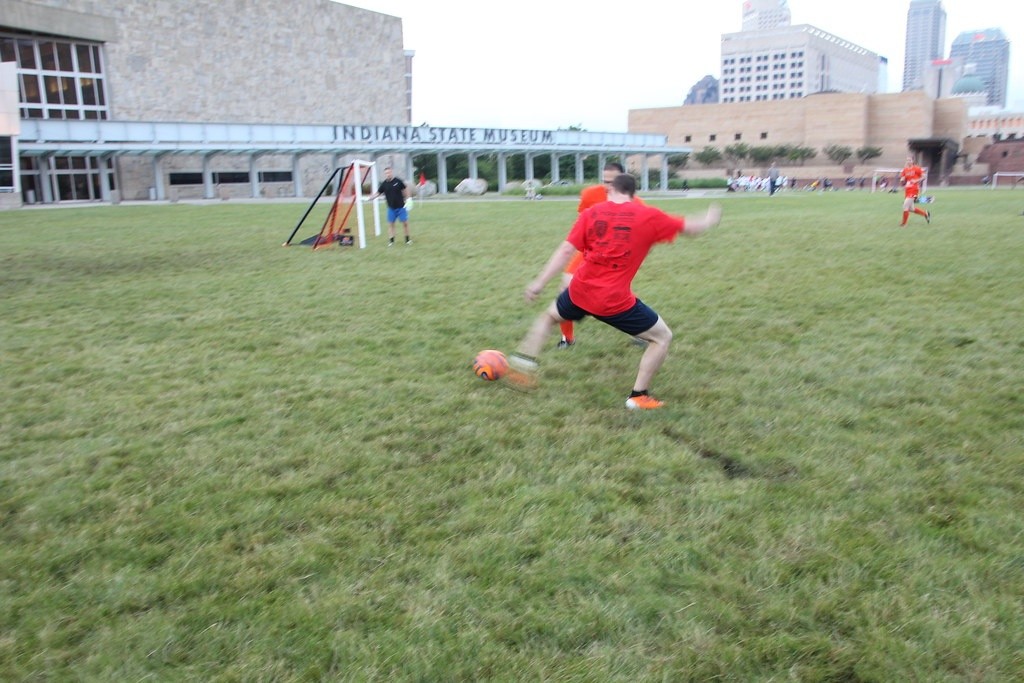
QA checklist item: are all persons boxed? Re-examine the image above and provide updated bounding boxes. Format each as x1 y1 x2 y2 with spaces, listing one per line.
680 161 899 196
365 167 411 245
555 164 645 348
899 156 930 227
508 173 721 410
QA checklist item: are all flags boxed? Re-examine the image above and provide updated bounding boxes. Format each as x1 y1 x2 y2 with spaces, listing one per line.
419 171 426 188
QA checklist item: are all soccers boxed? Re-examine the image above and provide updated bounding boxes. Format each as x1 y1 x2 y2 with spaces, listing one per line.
473 349 509 381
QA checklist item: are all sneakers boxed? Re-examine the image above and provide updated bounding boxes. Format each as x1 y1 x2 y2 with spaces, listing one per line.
552 335 575 352
625 394 665 409
387 242 394 246
405 240 412 245
925 210 930 224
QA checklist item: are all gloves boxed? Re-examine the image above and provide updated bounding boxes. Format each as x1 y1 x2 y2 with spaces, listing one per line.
361 196 370 201
403 197 413 211
